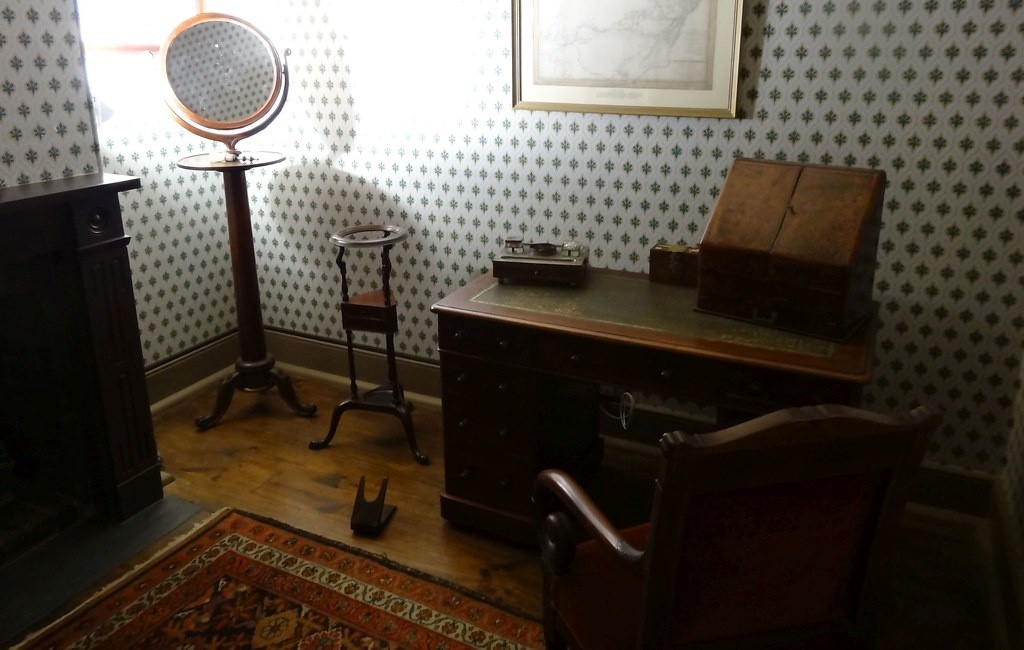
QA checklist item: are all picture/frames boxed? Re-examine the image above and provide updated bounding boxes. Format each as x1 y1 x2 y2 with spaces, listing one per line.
511 0 744 119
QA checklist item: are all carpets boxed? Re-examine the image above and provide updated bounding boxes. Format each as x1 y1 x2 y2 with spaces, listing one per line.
16 508 571 650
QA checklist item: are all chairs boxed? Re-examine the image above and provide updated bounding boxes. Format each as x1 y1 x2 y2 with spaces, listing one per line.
530 403 943 650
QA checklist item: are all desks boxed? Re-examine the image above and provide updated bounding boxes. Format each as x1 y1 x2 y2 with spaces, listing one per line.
177 151 318 430
430 267 873 555
